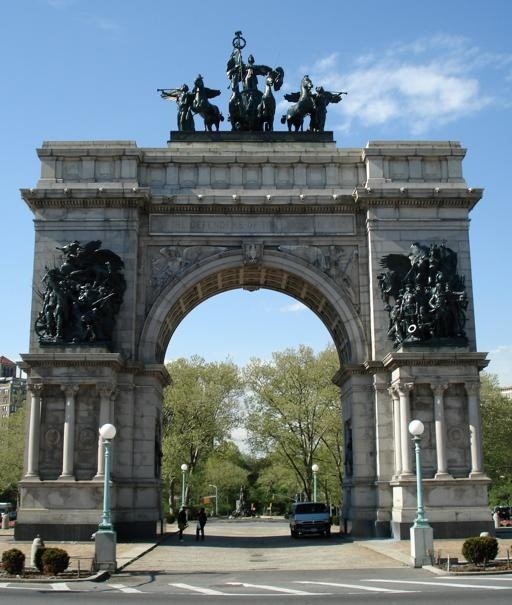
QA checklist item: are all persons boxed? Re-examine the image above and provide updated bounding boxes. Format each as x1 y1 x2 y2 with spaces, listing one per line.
379 238 470 345
163 84 199 129
178 504 189 542
38 239 125 346
245 54 262 90
309 84 339 133
198 507 208 540
493 507 500 527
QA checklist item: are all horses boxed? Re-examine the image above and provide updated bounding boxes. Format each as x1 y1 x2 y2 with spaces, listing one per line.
287 74 317 132
227 74 245 132
191 74 224 131
256 72 276 132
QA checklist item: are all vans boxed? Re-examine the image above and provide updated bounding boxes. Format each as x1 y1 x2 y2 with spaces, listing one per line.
288 502 333 538
0 503 14 521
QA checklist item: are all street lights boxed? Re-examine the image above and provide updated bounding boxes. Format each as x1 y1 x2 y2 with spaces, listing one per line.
311 465 319 503
408 420 431 528
98 423 117 529
209 485 218 516
181 463 188 506
